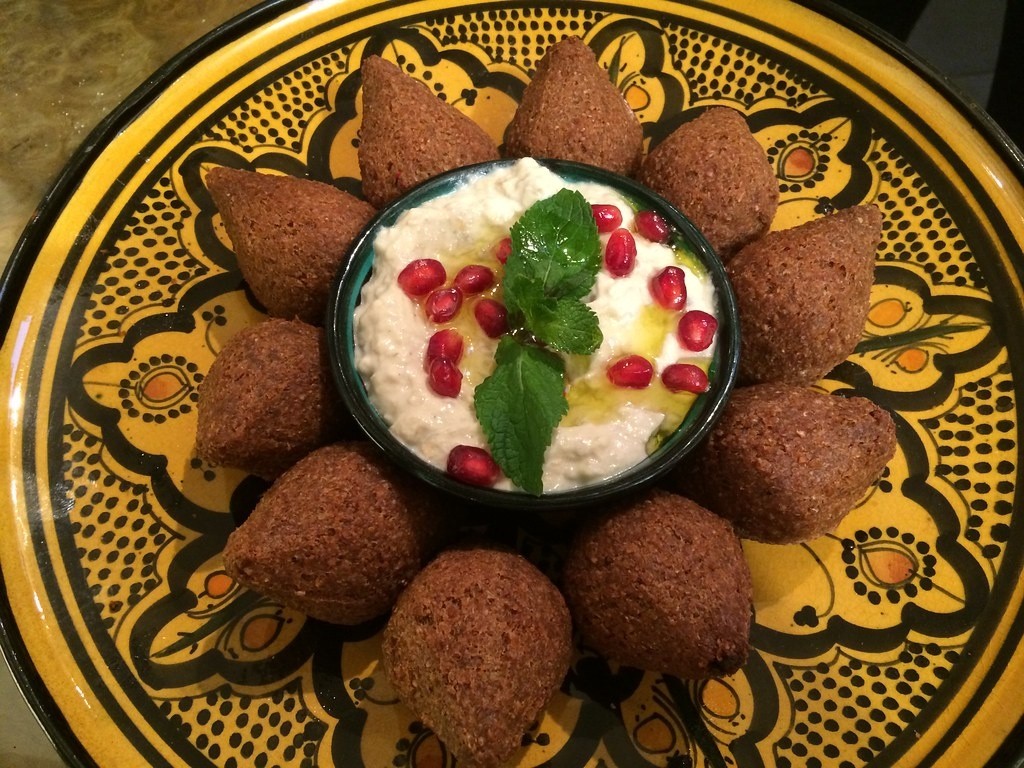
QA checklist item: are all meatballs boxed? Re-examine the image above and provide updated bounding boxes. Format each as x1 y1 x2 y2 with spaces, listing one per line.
638 107 778 256
723 206 882 385
222 437 438 623
196 318 344 474
361 57 499 205
700 382 893 546
204 167 376 318
556 489 747 674
503 38 642 177
382 545 573 767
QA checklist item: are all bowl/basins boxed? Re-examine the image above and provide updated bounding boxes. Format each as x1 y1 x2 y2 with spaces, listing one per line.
322 155 742 541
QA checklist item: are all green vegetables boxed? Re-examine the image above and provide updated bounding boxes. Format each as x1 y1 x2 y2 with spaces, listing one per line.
473 188 604 493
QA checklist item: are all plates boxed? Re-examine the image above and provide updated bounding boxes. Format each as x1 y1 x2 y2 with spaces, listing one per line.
0 1 1024 768
